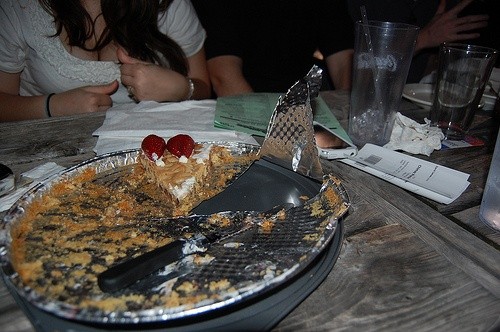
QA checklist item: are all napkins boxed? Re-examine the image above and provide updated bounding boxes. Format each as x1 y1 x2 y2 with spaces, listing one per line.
91 99 262 156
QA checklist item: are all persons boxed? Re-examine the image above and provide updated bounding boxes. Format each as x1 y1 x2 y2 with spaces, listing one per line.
206 0 489 97
0 0 210 123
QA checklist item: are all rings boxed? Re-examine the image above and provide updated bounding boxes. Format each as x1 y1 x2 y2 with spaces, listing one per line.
127 87 133 93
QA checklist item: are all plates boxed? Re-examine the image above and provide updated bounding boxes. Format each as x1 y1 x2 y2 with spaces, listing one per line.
401 83 434 110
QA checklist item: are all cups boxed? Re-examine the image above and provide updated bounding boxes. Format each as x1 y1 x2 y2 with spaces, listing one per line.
430 43 497 140
479 126 500 232
348 21 421 146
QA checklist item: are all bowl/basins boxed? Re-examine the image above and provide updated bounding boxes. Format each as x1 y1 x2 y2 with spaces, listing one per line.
480 93 498 111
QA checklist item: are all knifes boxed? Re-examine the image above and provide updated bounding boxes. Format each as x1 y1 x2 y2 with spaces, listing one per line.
97 203 293 292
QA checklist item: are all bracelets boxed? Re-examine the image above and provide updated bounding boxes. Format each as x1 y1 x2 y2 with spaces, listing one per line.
46 92 56 117
187 79 199 100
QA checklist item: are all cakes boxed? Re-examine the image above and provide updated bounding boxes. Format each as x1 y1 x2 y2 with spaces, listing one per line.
137 134 215 206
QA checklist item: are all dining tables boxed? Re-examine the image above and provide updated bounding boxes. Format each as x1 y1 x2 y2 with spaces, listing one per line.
0 83 500 332
